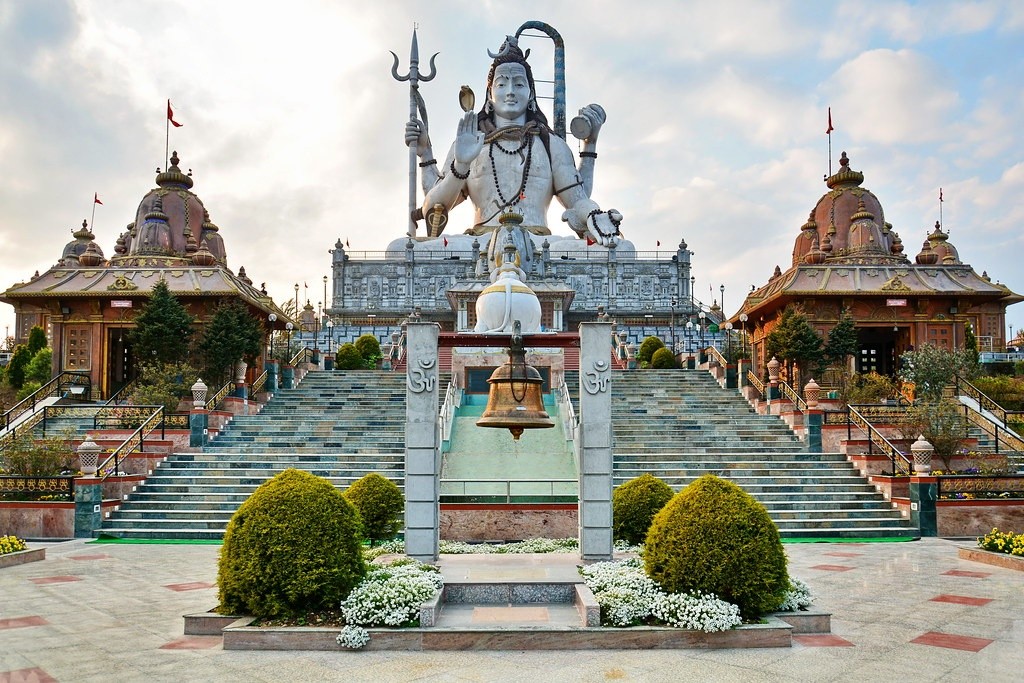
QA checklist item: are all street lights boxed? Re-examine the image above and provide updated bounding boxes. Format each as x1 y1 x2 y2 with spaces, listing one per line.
725 323 732 364
690 276 696 313
739 313 748 360
686 321 693 357
294 283 299 323
323 275 327 316
326 320 332 357
268 313 277 359
286 322 293 365
318 302 322 332
720 285 725 322
699 311 706 348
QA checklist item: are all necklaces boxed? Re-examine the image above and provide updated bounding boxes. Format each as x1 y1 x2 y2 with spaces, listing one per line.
489 134 533 206
493 134 528 155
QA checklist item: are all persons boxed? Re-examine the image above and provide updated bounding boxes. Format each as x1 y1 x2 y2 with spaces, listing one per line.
385 34 635 260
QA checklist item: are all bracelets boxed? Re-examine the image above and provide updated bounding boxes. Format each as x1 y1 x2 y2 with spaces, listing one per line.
587 208 620 237
579 152 597 158
450 159 470 180
419 159 437 167
